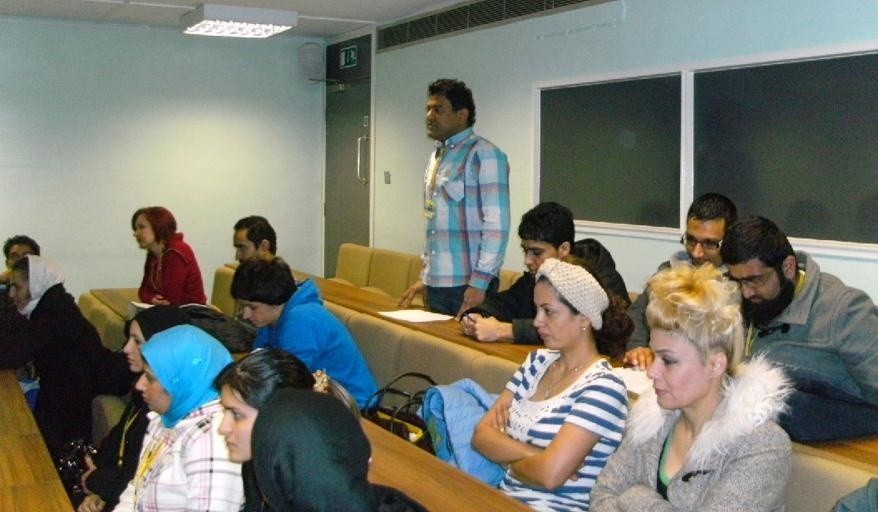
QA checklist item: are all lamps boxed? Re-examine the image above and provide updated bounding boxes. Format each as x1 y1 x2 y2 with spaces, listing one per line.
179 1 298 40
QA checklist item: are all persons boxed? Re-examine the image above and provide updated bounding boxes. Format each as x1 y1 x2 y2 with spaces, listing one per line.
397 79 511 320
458 201 637 354
1 255 111 461
721 217 878 405
590 262 793 512
212 346 360 512
233 215 296 335
472 256 631 512
1 235 40 314
252 387 430 512
131 207 208 306
622 194 738 372
111 325 246 512
75 304 196 512
231 252 380 415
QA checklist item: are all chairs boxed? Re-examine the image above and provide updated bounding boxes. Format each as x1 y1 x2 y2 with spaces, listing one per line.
205 265 238 313
330 241 375 288
354 250 426 297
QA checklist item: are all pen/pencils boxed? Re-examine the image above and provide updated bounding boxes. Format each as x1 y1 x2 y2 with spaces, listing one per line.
464 313 477 324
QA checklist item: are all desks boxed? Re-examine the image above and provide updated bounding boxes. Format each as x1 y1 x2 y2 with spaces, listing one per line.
78 288 538 512
0 357 74 512
225 262 874 494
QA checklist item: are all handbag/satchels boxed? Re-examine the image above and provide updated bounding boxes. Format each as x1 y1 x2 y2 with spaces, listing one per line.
359 371 439 457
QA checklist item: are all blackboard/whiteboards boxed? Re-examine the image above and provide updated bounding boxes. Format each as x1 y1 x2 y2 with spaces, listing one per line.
689 50 878 249
534 68 686 234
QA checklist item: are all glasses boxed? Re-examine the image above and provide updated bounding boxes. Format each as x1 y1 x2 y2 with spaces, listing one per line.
728 268 777 289
679 232 723 250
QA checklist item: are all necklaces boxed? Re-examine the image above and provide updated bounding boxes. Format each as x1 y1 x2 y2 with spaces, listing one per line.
544 355 600 401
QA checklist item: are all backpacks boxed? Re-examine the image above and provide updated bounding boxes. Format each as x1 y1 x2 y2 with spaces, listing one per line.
181 305 254 353
